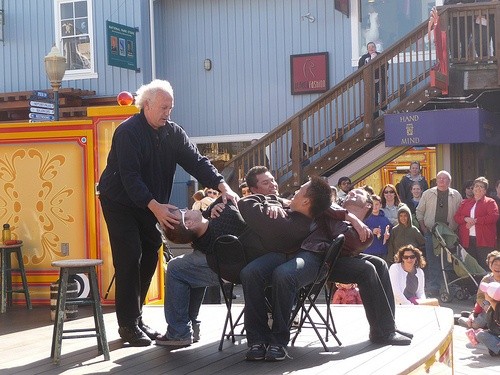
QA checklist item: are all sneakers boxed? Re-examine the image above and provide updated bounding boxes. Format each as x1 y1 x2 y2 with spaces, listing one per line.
246 344 268 361
156 331 194 346
266 345 287 361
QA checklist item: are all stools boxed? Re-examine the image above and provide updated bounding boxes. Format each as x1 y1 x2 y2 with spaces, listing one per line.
0 244 32 313
50 259 110 368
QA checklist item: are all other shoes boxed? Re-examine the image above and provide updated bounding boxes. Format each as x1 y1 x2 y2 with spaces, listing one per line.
118 325 151 345
139 324 159 339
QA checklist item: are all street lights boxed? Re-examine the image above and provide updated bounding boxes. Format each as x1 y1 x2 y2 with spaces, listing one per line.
41 42 68 122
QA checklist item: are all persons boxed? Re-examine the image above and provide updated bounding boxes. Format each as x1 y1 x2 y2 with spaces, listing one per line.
155 166 413 361
127 41 132 55
290 132 317 168
248 139 270 170
98 78 241 343
444 0 496 64
388 161 500 359
358 42 389 119
112 38 117 51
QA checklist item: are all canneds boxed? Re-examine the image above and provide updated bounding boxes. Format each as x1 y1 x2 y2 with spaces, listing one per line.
465 328 479 345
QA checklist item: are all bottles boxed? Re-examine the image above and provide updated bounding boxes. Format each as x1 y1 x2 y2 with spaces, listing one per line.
2 224 11 244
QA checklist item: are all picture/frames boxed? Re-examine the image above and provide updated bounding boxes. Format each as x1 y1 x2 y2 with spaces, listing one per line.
290 52 329 95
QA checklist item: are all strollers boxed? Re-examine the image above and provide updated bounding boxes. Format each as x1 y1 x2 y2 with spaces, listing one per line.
428 220 490 304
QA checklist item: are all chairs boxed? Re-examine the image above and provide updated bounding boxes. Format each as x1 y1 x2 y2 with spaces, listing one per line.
213 234 345 351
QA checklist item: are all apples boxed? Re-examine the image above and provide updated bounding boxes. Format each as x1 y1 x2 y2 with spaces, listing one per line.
117 91 133 105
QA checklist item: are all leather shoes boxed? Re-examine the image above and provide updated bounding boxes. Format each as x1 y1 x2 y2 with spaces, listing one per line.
395 328 414 338
370 331 411 345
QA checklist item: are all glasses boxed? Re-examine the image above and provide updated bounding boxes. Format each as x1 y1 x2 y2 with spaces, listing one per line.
474 184 486 188
242 189 250 194
342 182 350 185
403 254 416 259
384 190 394 194
179 209 189 229
208 191 217 195
